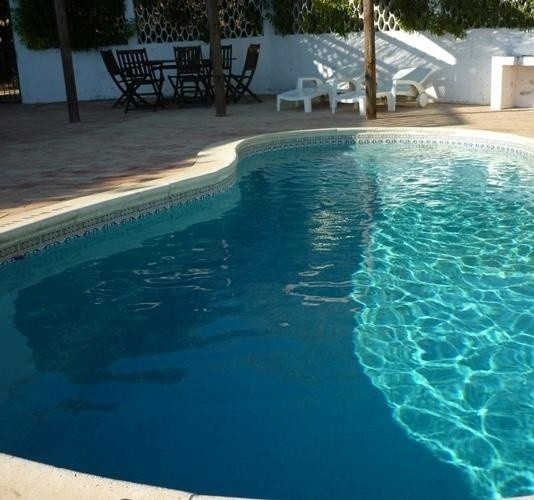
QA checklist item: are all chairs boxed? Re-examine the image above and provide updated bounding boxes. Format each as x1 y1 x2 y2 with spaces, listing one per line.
276 66 436 116
98 43 262 114
